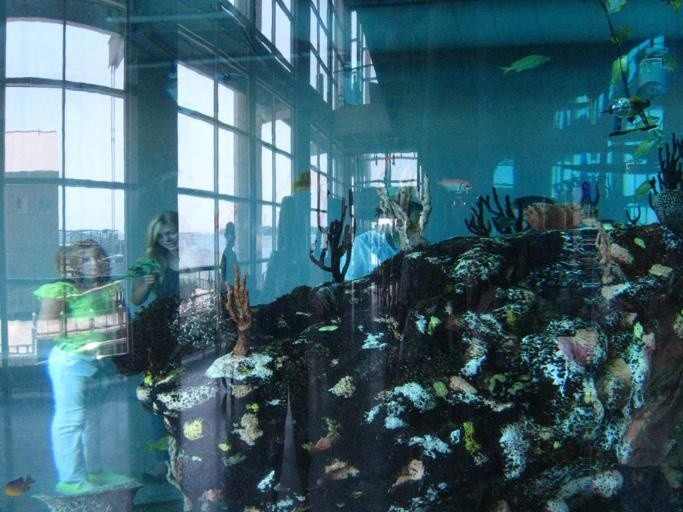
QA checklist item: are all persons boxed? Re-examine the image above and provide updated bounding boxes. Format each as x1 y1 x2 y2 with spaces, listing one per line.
340 195 424 280
221 223 239 281
258 195 310 479
130 212 178 306
33 240 133 495
